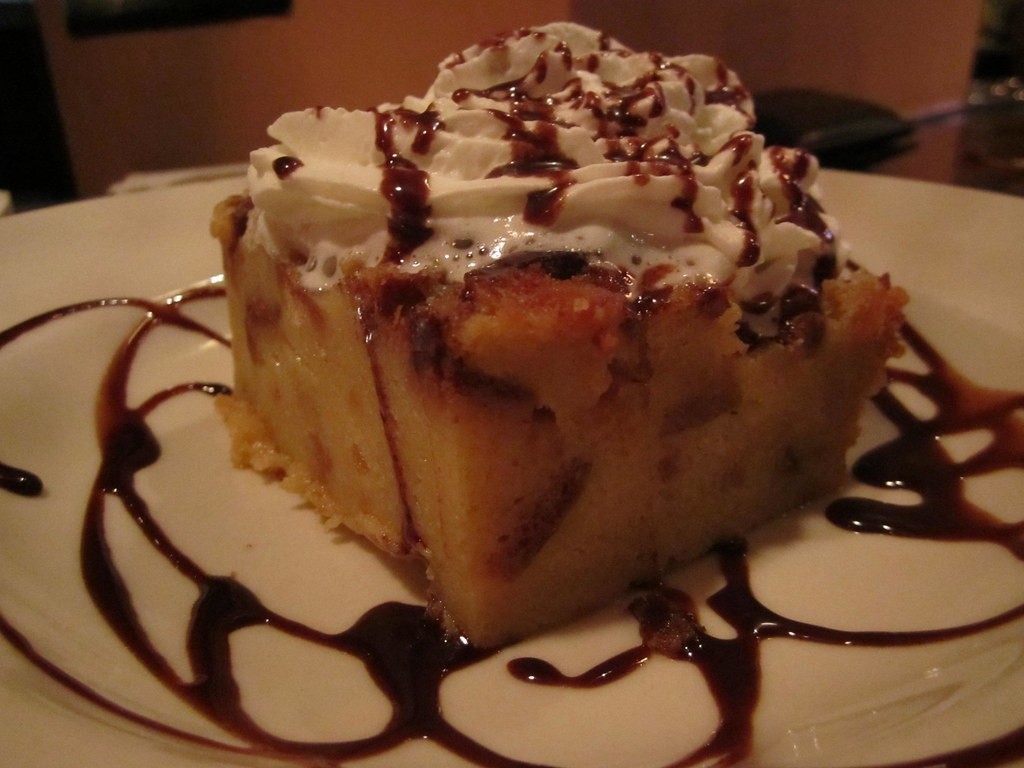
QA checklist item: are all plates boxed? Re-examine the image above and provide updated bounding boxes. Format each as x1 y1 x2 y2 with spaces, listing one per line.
0 170 1024 768
753 86 907 170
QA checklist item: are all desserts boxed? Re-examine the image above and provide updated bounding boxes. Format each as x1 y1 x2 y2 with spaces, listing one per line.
208 24 908 652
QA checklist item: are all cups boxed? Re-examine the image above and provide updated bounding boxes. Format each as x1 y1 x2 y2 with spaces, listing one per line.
0 0 78 215
